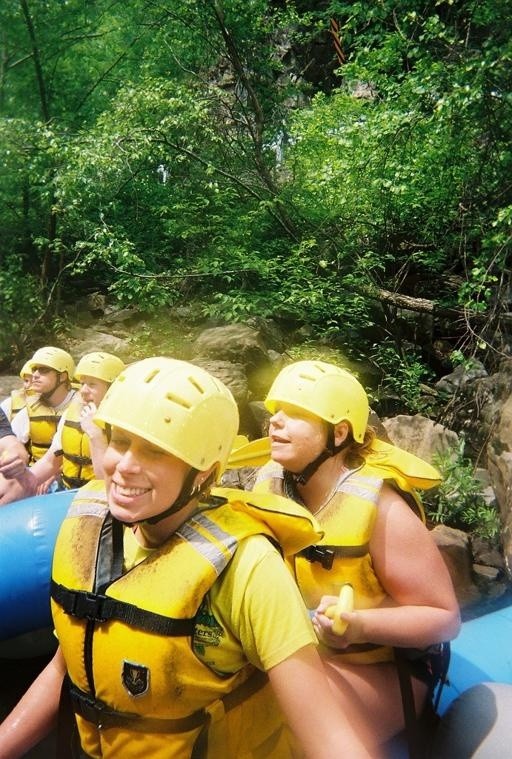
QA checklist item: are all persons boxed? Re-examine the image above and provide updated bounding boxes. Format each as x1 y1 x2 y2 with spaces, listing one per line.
1 405 30 482
244 361 462 758
12 346 80 466
1 358 40 427
0 351 127 507
0 357 373 758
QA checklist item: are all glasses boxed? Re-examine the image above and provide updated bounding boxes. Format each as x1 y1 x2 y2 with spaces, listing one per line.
31 365 52 374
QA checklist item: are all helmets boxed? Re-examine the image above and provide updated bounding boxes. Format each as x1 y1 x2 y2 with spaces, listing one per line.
74 351 124 384
29 346 73 380
263 359 370 448
19 359 31 376
95 355 241 484
